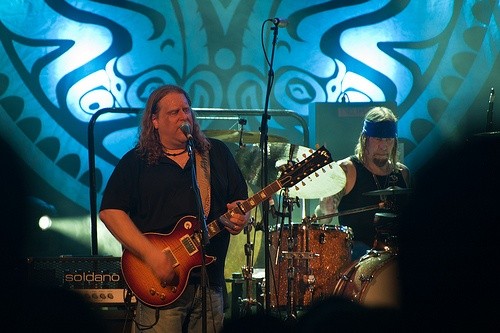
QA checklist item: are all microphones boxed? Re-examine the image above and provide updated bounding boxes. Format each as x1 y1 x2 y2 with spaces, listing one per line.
180 122 193 140
270 17 288 27
487 87 495 132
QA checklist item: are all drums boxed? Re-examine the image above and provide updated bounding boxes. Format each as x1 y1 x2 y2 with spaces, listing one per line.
334 250 397 307
269 223 354 312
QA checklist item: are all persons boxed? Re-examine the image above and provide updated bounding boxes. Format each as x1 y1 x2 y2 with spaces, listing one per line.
99 84 251 333
314 106 409 263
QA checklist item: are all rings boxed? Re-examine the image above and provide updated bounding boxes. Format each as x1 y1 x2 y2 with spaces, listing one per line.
234 226 236 229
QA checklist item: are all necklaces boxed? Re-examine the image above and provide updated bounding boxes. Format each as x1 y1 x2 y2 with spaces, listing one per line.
164 149 186 156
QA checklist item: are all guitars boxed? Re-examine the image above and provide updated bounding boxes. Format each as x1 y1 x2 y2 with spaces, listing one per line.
120 144 334 308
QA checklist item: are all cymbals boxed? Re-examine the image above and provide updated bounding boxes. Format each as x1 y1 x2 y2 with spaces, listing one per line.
362 186 411 196
201 130 289 143
234 144 347 199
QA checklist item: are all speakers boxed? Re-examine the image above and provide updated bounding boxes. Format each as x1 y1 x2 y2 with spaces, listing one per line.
308 101 397 162
